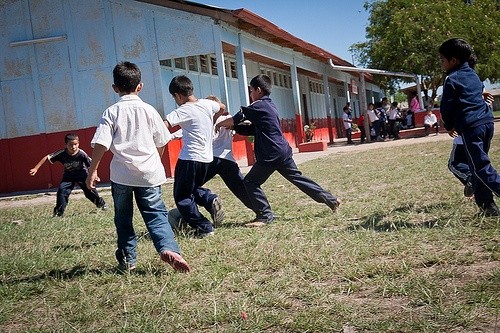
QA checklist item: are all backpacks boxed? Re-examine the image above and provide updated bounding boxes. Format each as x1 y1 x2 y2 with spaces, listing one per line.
375 108 387 124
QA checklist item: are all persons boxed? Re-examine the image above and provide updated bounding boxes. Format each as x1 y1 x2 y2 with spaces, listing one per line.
202 96 266 222
343 102 366 144
163 75 225 237
411 89 441 111
438 38 500 217
30 134 109 217
424 109 439 136
367 97 413 141
86 61 192 272
215 74 340 227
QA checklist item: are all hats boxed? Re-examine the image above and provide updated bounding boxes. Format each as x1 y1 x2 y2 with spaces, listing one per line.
381 97 389 102
392 102 398 107
425 108 433 112
368 103 375 107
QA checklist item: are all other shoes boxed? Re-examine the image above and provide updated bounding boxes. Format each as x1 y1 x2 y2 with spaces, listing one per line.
464 181 474 196
245 221 268 226
371 135 398 141
102 203 109 211
210 197 225 228
348 141 354 144
192 230 217 239
117 262 136 272
332 198 343 214
160 250 191 274
473 202 500 222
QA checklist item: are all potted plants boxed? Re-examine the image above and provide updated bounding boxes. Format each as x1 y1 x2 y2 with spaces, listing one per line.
299 120 327 152
351 123 362 139
